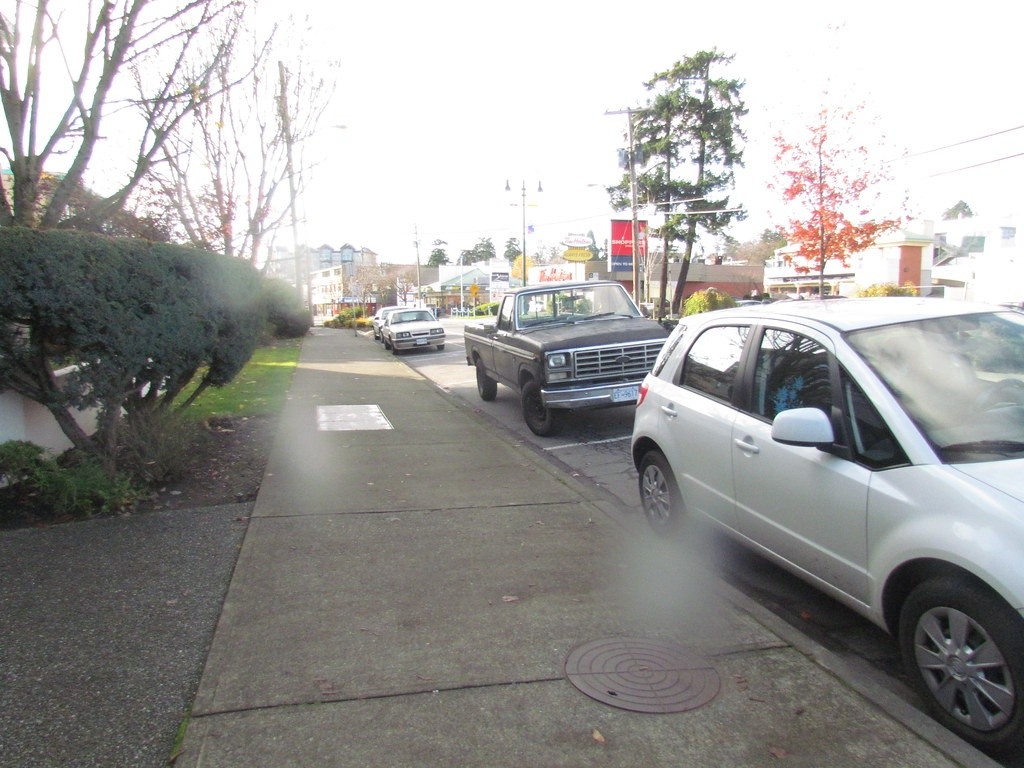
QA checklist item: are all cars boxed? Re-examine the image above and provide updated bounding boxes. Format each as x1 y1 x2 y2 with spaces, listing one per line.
372 306 409 340
381 308 447 355
639 297 670 316
735 300 762 307
631 296 1024 750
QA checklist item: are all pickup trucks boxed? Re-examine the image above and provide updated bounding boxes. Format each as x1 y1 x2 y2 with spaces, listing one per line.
464 280 670 436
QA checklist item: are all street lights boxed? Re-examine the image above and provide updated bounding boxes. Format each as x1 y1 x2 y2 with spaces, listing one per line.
505 179 543 315
